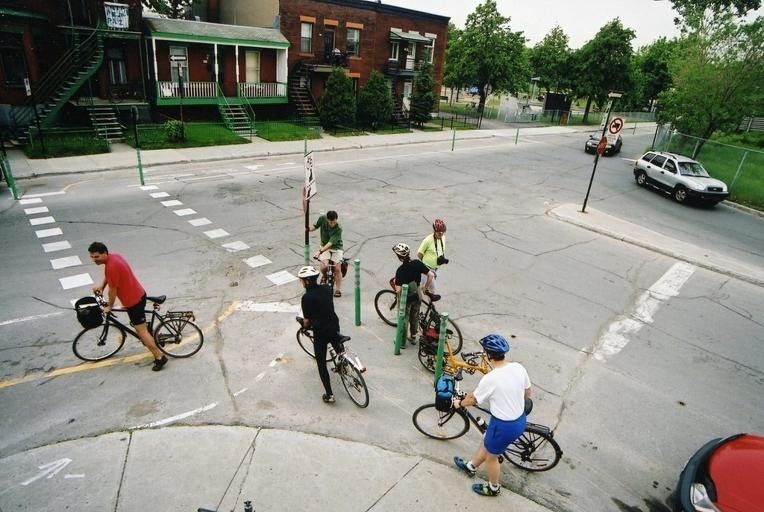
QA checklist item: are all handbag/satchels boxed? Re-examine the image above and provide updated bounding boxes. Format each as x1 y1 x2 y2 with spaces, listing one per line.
437 375 453 398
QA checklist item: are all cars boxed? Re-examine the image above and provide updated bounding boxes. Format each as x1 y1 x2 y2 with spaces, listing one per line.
676 432 764 512
585 129 623 156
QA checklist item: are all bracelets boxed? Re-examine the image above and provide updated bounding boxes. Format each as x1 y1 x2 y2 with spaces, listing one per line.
458 399 463 407
318 249 323 255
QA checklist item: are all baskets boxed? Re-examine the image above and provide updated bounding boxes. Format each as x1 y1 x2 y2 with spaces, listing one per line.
435 374 453 411
75 296 103 329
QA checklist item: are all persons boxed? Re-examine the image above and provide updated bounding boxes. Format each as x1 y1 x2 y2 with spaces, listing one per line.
454 334 533 497
88 242 168 371
416 219 447 295
296 265 347 403
308 211 345 297
392 243 434 349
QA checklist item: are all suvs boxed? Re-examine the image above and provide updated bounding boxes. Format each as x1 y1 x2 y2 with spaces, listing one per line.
633 150 729 206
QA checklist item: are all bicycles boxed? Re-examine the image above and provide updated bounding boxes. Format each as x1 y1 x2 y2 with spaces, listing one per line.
416 325 493 380
374 275 463 360
412 372 562 473
294 313 370 409
71 287 205 363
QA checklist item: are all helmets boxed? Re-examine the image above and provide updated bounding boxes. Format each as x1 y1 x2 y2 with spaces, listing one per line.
479 334 509 353
392 243 410 258
297 266 320 279
433 218 446 233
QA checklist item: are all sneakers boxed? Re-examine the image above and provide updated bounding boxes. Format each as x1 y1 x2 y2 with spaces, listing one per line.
408 336 416 345
333 291 341 297
323 393 336 402
472 482 501 496
152 355 168 371
394 338 406 349
454 456 476 477
